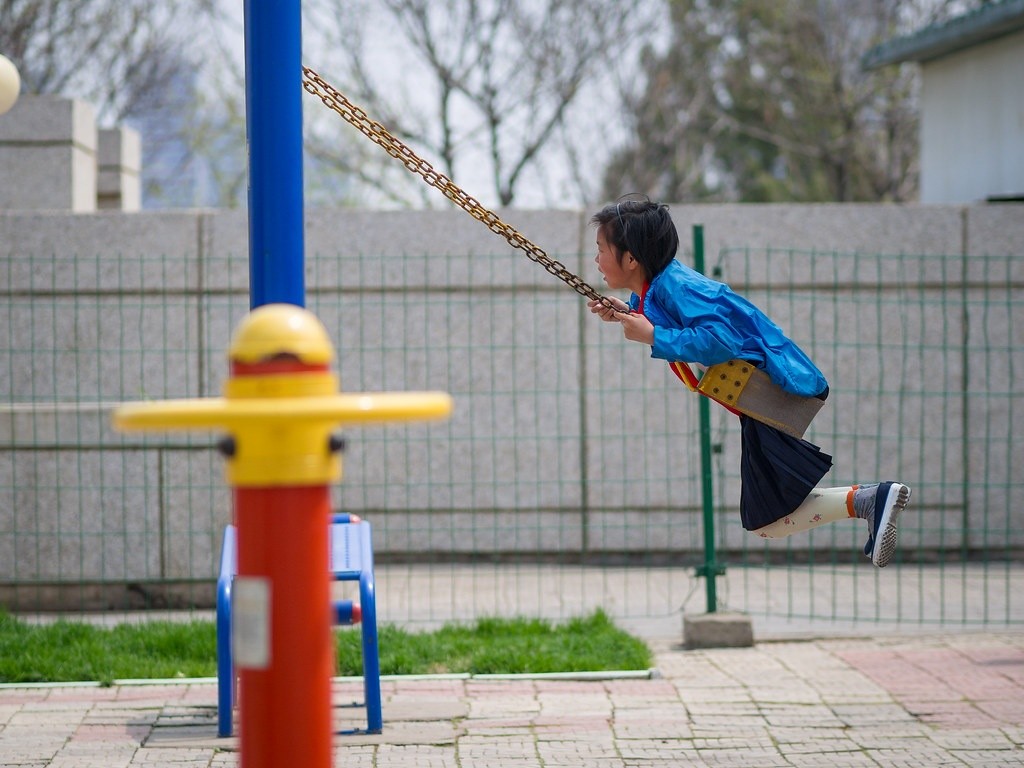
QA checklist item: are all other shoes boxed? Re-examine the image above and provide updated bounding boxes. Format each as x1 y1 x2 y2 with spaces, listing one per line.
864 481 912 558
870 483 908 568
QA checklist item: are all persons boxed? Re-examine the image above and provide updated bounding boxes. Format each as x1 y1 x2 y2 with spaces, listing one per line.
586 192 912 568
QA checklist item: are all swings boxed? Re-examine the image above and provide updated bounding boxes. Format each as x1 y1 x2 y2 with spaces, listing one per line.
303 65 824 441
